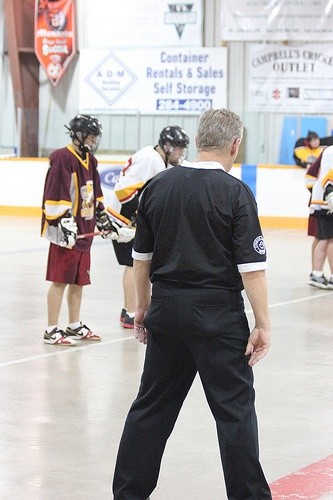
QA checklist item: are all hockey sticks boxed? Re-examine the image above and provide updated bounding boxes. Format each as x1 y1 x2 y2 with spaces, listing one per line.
114 220 136 244
43 224 109 247
325 191 333 214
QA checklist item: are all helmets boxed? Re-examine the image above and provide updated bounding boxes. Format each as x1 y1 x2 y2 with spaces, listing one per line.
64 114 103 142
159 124 190 147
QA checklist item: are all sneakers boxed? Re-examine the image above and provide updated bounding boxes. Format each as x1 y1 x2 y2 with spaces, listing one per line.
66 324 102 341
308 272 333 290
43 327 77 346
120 308 135 329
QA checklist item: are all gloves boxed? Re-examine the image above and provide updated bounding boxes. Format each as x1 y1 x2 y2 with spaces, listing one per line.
59 217 78 246
96 210 121 240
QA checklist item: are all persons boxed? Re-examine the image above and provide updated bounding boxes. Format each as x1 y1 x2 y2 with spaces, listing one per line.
303 144 333 289
112 108 272 500
40 115 100 346
293 130 333 169
105 125 190 328
